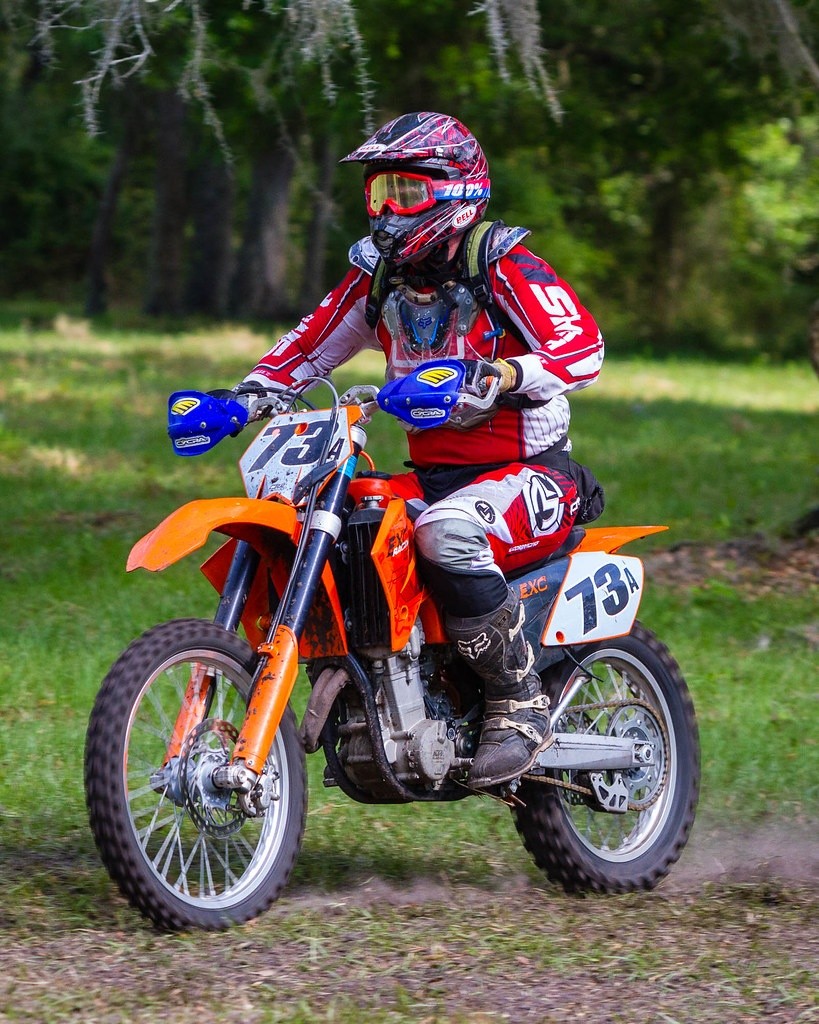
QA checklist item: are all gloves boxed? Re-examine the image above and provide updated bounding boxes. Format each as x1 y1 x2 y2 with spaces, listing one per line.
456 357 513 397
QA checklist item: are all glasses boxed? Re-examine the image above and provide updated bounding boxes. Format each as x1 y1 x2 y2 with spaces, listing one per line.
366 173 437 215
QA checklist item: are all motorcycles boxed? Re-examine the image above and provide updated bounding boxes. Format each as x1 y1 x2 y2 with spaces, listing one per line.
83 360 701 935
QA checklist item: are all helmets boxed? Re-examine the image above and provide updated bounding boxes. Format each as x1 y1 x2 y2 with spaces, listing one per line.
339 112 489 267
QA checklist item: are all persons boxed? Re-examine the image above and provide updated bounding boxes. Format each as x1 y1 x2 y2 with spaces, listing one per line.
163 108 609 791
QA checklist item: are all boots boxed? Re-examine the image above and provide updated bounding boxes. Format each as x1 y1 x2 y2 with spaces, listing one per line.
322 733 342 786
440 584 558 790
